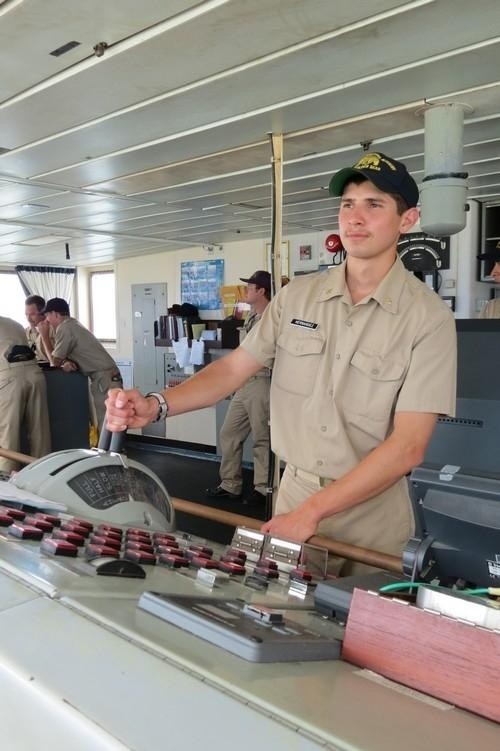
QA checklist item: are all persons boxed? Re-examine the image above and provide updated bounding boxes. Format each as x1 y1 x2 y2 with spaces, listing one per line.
104 151 460 576
0 316 52 477
205 271 275 505
35 298 126 455
475 239 500 319
23 294 58 361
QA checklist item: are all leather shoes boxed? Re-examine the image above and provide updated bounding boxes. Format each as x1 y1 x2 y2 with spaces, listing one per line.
201 485 234 497
245 490 265 501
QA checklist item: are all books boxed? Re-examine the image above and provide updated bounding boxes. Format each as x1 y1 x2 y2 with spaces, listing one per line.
159 312 206 342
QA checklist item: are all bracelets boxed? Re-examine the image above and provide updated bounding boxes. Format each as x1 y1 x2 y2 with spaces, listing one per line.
145 392 168 423
61 359 68 364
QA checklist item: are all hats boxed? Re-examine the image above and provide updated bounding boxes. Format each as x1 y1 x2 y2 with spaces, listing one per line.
40 298 69 314
329 151 420 212
240 270 271 289
